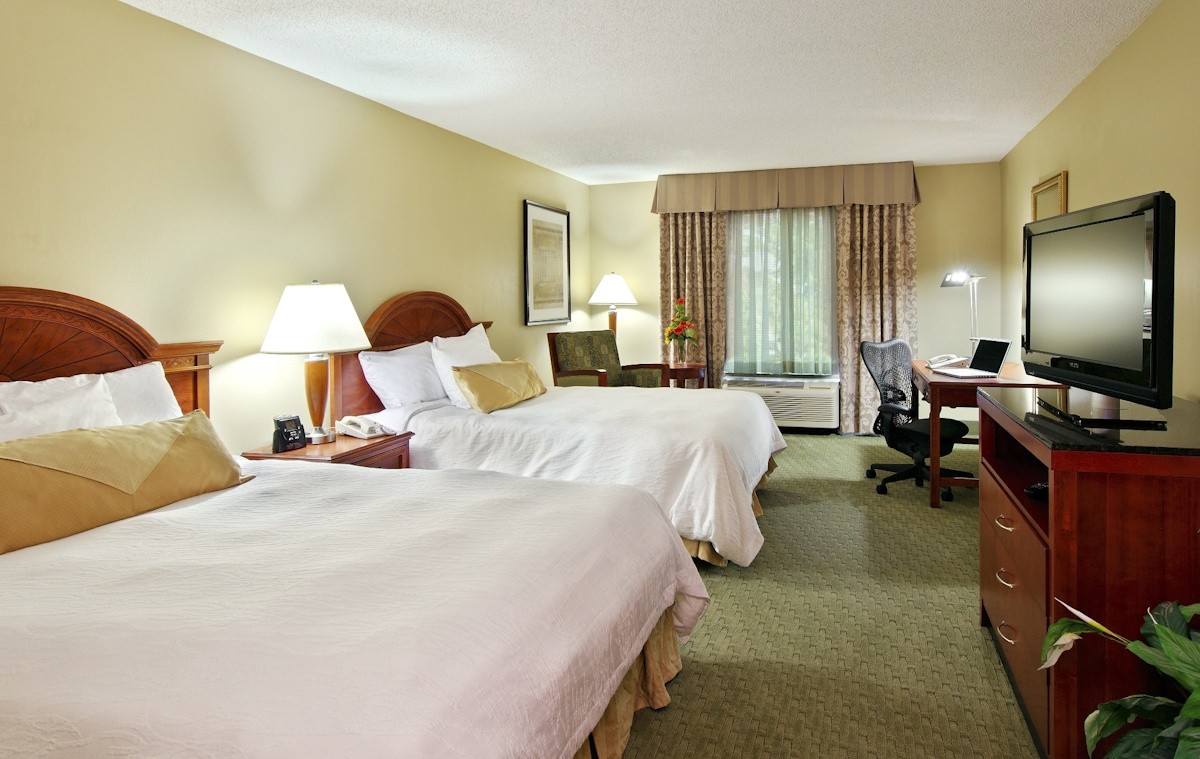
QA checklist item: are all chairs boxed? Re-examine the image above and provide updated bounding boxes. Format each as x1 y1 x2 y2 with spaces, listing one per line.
861 337 977 502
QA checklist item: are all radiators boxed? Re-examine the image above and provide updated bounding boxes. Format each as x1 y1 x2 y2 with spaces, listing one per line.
721 372 840 436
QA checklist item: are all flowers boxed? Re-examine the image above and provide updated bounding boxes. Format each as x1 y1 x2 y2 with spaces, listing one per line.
664 297 701 363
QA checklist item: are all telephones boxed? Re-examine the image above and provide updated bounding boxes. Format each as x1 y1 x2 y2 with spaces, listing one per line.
923 354 969 371
334 415 397 441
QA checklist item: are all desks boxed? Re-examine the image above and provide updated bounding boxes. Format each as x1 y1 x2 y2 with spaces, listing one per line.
668 362 707 389
910 358 1071 509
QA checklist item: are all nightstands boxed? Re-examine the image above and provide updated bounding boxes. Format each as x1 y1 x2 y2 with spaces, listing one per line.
240 430 415 469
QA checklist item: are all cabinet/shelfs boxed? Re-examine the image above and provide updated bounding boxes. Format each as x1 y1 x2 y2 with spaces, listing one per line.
974 390 1200 759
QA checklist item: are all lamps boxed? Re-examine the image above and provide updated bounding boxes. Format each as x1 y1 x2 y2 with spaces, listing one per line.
941 270 986 356
258 280 372 445
587 272 639 338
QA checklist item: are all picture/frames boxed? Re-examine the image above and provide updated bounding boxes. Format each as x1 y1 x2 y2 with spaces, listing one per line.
1031 170 1068 221
523 199 572 326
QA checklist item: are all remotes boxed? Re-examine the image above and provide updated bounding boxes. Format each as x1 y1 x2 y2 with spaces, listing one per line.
1024 479 1049 498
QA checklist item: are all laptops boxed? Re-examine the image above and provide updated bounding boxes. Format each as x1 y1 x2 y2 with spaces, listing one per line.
933 336 1011 378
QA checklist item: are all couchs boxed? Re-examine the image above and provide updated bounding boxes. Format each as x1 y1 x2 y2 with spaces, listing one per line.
547 329 670 388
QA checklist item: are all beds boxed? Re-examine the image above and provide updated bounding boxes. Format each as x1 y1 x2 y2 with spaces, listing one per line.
1 453 712 759
356 384 789 570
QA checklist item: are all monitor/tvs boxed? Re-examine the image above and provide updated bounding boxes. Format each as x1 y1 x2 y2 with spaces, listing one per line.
1022 190 1176 409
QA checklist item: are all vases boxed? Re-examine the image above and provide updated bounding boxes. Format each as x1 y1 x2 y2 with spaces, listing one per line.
676 338 687 368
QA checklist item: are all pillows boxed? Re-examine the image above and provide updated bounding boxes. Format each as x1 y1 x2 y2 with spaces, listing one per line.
0 408 256 555
451 357 547 414
103 361 184 424
431 322 503 410
358 341 448 409
0 373 122 440
